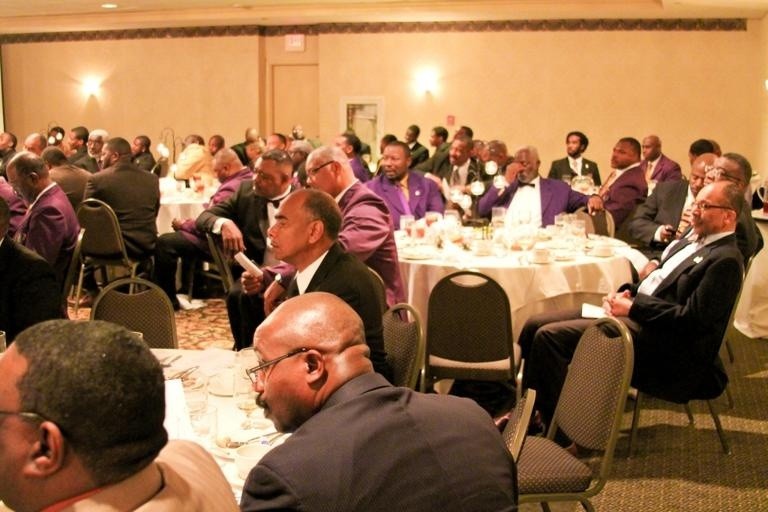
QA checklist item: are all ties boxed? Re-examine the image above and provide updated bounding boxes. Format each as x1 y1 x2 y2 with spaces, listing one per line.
286 279 298 297
393 180 411 215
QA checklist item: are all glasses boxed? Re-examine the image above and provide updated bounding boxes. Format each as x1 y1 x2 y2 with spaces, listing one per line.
704 166 743 182
692 201 731 211
309 159 333 174
246 347 308 384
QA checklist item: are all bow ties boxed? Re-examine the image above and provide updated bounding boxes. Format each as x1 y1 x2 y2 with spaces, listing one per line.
266 198 279 208
518 183 535 187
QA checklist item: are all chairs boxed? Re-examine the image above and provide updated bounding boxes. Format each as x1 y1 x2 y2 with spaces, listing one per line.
573 206 615 239
382 303 424 389
369 266 387 314
205 232 235 295
89 278 178 351
719 252 755 362
629 387 734 455
502 389 537 464
512 316 634 512
74 198 154 319
425 271 525 419
63 228 86 298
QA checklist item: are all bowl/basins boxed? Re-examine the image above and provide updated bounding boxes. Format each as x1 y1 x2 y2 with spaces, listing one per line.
187 347 275 477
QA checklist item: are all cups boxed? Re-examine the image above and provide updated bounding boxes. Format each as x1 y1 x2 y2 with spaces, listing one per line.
561 175 599 195
176 174 205 200
401 206 614 265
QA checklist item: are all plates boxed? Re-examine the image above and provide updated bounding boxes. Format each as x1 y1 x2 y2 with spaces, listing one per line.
165 371 203 390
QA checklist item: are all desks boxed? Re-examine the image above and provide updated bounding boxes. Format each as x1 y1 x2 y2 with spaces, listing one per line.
155 175 222 237
749 207 768 229
151 347 293 504
392 228 649 342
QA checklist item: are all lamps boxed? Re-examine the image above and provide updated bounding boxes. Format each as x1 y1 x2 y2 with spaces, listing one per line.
39 119 63 149
154 126 187 166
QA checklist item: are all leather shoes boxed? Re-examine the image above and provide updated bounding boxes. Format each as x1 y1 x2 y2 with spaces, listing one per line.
494 408 543 432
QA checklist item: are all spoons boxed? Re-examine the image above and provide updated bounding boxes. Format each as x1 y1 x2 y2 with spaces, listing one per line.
160 354 183 367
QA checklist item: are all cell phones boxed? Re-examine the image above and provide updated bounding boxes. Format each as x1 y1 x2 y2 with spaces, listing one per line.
666 227 676 237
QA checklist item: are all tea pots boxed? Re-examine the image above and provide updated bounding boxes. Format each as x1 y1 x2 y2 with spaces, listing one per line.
757 179 768 217
